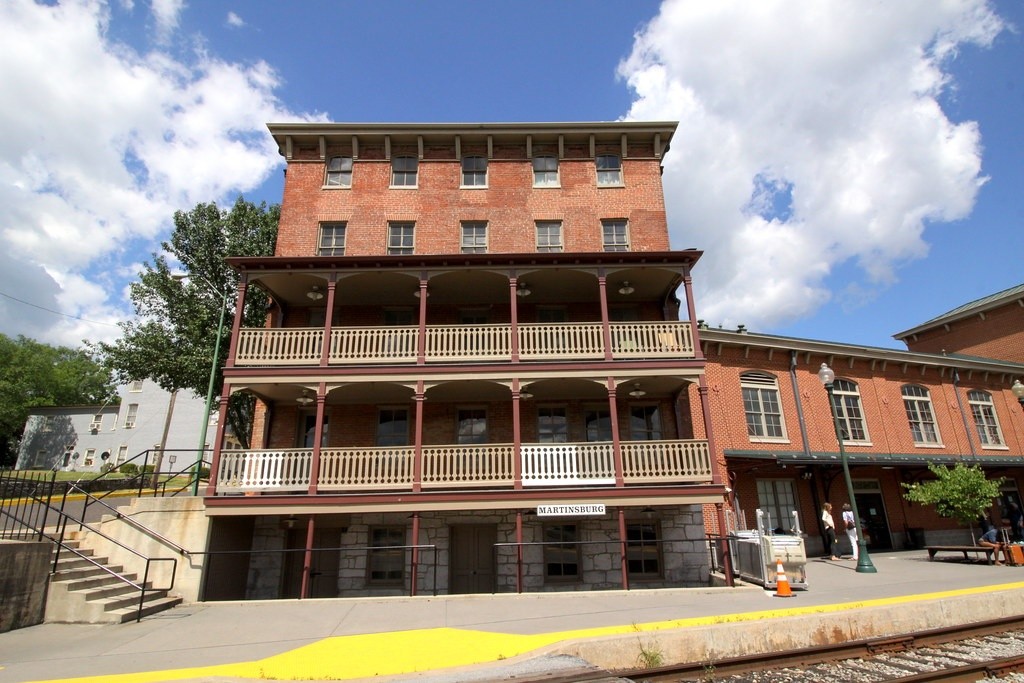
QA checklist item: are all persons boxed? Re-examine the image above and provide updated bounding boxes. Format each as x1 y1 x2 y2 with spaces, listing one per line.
821 501 859 561
977 522 1007 567
999 501 1024 541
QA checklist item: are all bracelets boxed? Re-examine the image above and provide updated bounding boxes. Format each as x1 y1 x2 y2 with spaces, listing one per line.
999 541 1001 545
1003 542 1004 544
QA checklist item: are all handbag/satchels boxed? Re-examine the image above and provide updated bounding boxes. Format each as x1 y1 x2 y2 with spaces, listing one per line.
848 521 854 530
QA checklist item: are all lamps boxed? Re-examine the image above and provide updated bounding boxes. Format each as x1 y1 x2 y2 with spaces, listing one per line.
410 396 428 400
641 507 655 516
296 390 313 405
306 286 324 300
524 510 537 516
618 281 635 295
516 283 531 296
285 515 298 527
414 286 430 297
407 514 422 519
629 383 646 399
520 385 533 401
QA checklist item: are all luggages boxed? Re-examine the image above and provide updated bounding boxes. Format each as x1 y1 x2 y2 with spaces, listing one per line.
1001 528 1024 567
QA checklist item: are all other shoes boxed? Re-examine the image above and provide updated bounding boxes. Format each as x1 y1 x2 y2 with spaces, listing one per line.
848 557 857 561
829 555 841 561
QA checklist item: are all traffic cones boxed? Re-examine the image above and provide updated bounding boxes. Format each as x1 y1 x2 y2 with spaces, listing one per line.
770 559 799 597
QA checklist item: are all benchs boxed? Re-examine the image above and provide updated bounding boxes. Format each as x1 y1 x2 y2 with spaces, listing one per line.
922 545 996 564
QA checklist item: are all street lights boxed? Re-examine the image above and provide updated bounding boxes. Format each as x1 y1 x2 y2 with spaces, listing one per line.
816 362 877 574
